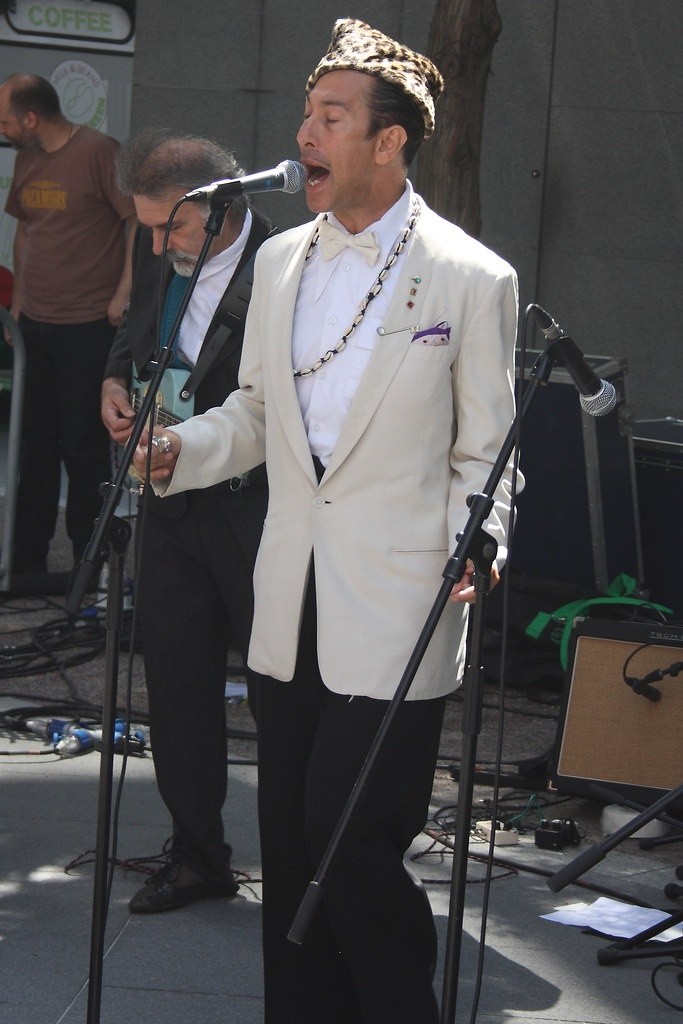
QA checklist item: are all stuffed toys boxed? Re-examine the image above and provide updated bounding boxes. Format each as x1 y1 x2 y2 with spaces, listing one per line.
412 321 451 345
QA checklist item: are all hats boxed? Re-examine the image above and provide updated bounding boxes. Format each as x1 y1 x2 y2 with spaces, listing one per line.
306 17 446 141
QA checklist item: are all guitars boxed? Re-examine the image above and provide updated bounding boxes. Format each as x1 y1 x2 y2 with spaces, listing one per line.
111 359 249 517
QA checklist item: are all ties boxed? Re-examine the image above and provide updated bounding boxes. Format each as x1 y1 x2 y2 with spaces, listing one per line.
160 273 195 371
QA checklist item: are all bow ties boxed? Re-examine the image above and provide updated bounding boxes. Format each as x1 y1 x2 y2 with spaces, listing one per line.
319 221 379 267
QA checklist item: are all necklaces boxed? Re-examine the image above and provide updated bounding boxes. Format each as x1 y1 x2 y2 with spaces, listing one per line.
292 193 421 378
68 123 72 140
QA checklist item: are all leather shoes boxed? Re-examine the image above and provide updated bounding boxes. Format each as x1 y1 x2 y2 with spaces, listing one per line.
128 847 236 914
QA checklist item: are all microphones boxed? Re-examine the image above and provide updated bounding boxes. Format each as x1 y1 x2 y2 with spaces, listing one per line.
184 160 307 202
625 676 662 702
532 304 617 417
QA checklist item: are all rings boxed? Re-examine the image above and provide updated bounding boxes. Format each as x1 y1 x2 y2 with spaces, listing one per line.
153 436 172 453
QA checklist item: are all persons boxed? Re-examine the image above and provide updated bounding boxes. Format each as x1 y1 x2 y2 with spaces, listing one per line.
0 263 15 369
0 75 138 574
101 128 279 914
131 18 525 1024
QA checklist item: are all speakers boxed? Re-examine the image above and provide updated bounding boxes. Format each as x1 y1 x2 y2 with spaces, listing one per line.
547 417 683 814
